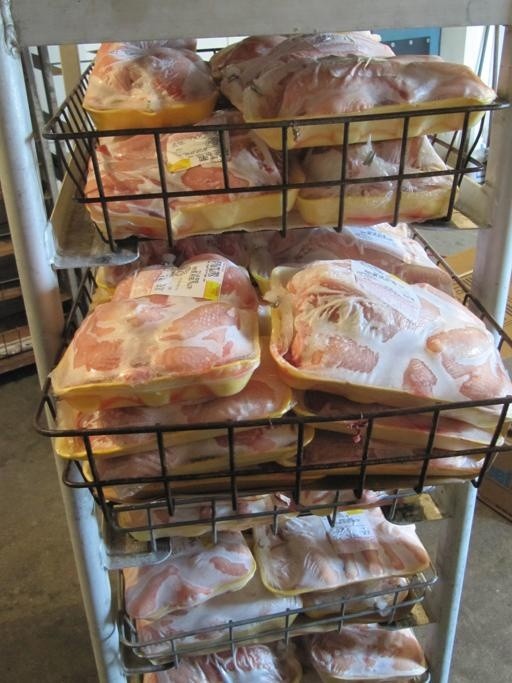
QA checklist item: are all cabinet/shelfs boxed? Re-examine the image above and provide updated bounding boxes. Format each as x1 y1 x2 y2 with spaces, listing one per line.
0 0 511 681
